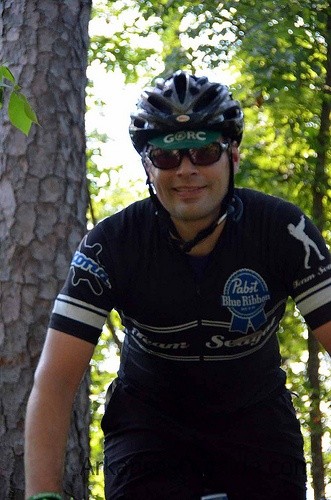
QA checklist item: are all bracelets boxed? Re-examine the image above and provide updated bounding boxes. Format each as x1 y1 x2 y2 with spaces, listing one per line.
23 490 64 499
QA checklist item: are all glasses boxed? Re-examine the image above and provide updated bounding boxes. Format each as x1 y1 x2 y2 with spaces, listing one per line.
140 142 229 170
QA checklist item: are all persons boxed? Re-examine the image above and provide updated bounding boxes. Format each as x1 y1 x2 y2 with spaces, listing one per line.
20 74 331 499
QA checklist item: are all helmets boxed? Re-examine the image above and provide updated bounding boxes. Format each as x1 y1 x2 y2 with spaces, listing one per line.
128 71 244 156
